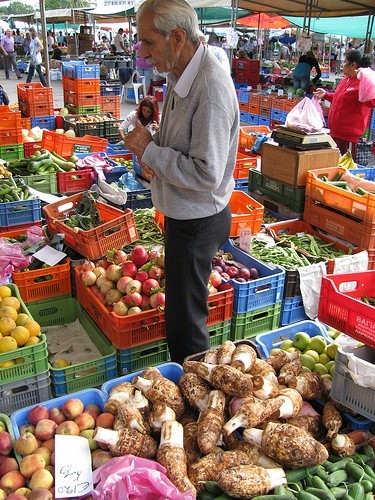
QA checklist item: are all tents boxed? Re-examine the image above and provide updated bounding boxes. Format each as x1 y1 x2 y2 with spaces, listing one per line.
0 0 375 60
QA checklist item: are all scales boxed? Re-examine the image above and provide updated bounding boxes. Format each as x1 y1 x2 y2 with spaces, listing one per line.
272 125 338 151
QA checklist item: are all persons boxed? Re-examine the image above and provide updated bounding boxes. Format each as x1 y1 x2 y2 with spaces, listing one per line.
314 50 375 163
25 28 47 88
0 85 9 106
124 0 239 365
95 28 153 98
207 33 375 74
118 96 162 141
0 28 70 80
292 51 320 93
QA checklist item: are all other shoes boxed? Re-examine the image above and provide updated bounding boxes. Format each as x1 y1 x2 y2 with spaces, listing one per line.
18 76 23 79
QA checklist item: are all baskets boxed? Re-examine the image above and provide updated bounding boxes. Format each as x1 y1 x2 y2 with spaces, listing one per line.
0 53 374 473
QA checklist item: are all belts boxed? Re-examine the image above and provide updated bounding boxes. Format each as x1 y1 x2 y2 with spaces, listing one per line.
6 51 14 53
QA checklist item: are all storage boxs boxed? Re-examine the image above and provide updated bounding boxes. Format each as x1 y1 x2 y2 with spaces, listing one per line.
1 57 375 488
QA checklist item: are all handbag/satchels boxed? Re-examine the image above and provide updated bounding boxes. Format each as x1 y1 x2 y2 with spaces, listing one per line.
30 53 37 67
309 94 327 127
284 96 323 134
355 67 375 102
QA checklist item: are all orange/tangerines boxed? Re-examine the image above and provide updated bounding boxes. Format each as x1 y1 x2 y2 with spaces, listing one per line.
55 359 69 368
0 285 41 369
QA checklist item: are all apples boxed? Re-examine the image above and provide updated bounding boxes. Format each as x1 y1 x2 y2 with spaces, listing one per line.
269 331 338 379
208 257 259 295
0 397 116 500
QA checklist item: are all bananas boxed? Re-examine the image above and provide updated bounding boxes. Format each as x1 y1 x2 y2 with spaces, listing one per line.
338 150 354 170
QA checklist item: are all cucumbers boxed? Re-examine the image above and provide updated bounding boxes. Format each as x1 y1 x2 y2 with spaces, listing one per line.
320 171 369 196
0 174 36 212
194 444 375 500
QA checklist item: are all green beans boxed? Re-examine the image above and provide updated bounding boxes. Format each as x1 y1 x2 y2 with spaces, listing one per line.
132 207 165 246
248 233 352 271
